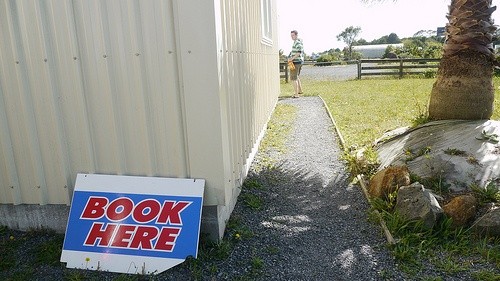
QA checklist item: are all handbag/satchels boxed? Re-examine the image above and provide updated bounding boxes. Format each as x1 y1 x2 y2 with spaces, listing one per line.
287 59 295 72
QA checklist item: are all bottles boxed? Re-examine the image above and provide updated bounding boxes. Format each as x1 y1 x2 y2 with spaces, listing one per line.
288 61 295 70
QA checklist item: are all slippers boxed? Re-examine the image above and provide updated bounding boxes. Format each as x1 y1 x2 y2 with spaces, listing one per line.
291 91 303 98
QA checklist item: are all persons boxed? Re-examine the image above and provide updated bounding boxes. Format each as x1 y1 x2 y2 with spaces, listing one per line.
287 31 305 98
